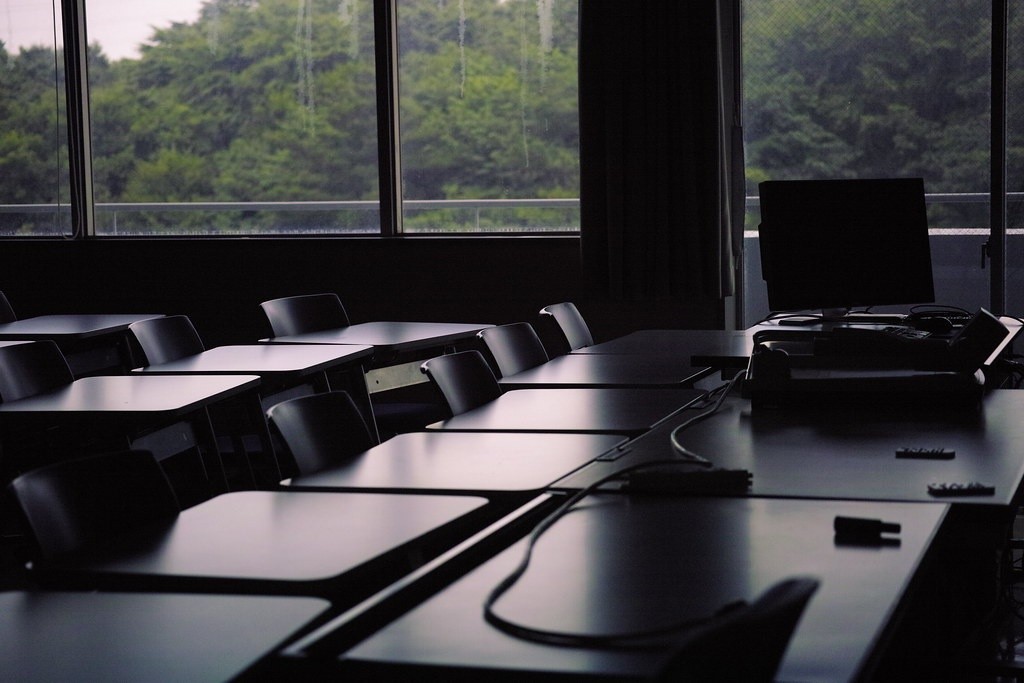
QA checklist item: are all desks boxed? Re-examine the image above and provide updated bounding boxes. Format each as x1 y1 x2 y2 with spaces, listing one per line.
748 310 1024 387
425 387 708 432
260 321 497 443
552 387 1024 683
0 590 331 683
0 375 261 493
568 329 757 358
0 314 166 376
132 344 380 488
341 494 952 683
279 431 630 494
497 355 712 384
56 491 489 584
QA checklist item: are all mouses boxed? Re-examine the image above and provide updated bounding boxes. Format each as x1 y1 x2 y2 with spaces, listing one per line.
925 317 954 334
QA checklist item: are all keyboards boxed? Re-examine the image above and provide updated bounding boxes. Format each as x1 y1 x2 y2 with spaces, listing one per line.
880 327 932 342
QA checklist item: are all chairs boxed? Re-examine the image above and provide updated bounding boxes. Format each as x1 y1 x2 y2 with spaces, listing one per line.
259 294 434 404
3 451 184 561
420 351 503 418
0 340 74 403
475 322 549 377
263 390 375 480
127 315 276 488
539 301 594 352
0 290 16 326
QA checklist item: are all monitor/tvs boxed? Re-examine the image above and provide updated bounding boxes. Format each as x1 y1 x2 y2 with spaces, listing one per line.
757 177 935 326
